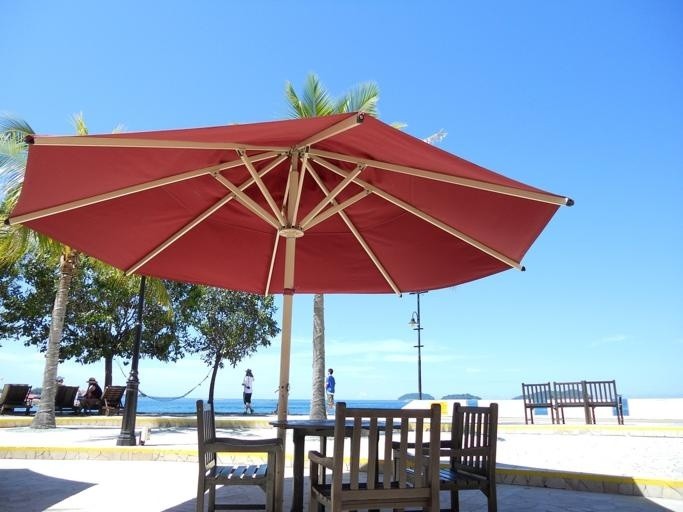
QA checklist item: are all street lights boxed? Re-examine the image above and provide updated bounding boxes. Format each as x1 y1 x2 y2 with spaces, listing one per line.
409 292 424 399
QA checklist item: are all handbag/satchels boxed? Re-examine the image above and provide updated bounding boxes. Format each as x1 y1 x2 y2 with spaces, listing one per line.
73 391 81 408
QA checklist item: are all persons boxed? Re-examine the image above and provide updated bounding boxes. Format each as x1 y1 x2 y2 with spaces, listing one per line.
57 375 65 385
71 376 102 416
242 368 254 414
324 368 336 409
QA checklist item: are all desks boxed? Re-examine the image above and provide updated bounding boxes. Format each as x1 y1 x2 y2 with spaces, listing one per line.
269 419 409 510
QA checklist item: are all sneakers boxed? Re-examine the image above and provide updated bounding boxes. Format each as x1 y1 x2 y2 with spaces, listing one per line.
243 410 247 414
250 409 254 414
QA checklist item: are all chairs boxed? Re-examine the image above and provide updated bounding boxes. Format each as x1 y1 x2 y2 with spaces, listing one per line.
391 402 500 512
195 397 286 512
308 400 441 511
0 384 33 416
582 379 625 425
53 384 79 414
552 381 590 424
78 385 127 415
521 382 557 425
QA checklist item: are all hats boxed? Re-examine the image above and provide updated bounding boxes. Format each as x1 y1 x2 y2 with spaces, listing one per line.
57 376 64 381
245 369 252 374
85 377 98 384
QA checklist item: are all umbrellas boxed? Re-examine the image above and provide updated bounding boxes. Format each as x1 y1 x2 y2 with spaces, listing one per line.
3 110 575 511
